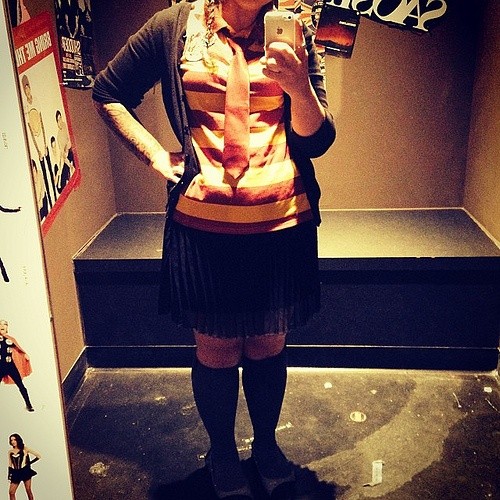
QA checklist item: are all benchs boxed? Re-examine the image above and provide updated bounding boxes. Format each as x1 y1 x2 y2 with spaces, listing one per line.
70 206 500 372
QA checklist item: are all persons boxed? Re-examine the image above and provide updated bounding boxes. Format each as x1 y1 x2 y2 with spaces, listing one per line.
8 434 41 500
21 75 76 220
1 319 35 412
90 0 338 500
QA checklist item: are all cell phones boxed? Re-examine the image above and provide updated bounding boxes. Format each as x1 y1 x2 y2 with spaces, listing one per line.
264 11 296 59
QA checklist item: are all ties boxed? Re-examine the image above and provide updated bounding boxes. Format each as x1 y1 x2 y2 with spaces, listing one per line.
221 27 257 180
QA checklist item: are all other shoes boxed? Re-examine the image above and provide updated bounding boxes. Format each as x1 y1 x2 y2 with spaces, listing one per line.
208 445 253 500
252 438 297 497
